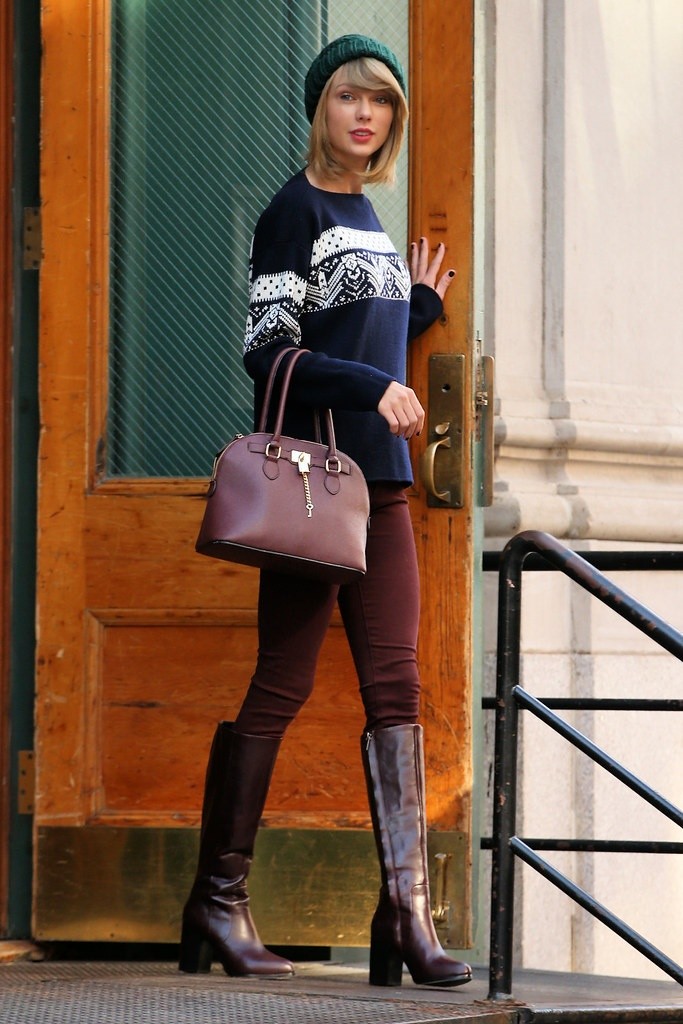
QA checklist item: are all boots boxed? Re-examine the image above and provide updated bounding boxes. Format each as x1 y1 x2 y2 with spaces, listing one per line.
359 724 472 988
178 720 295 982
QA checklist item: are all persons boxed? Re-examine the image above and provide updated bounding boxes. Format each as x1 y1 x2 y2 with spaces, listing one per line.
180 33 474 989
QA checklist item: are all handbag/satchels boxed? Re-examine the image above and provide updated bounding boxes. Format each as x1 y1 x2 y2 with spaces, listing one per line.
194 349 370 583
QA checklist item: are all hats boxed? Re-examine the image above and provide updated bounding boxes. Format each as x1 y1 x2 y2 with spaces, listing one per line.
304 34 407 127
